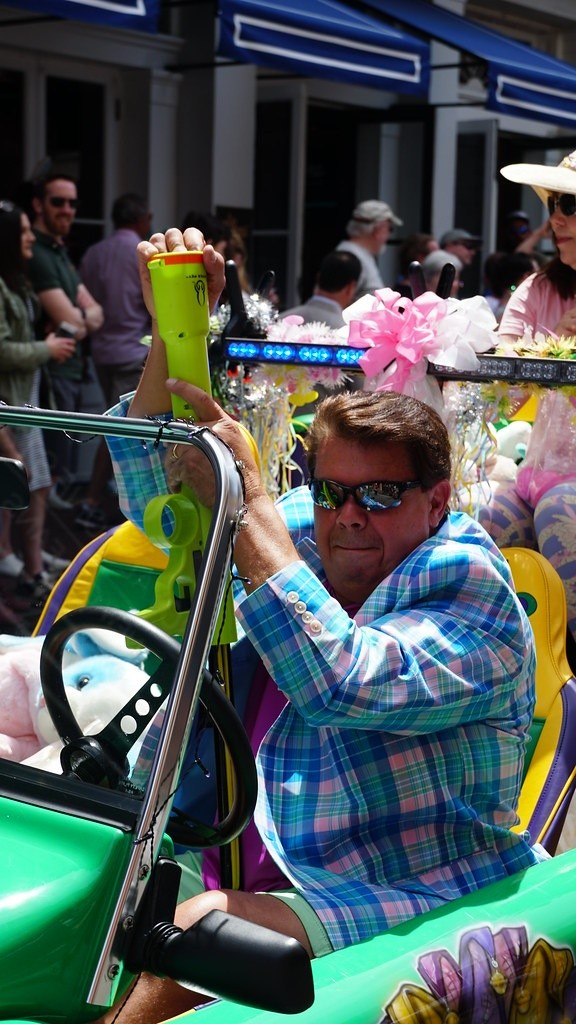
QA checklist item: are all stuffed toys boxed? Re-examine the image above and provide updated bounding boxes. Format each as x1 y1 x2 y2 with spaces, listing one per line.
1 625 164 786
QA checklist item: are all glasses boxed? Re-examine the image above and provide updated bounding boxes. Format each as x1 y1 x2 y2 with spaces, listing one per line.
49 196 79 210
547 195 576 216
452 239 477 251
308 476 423 512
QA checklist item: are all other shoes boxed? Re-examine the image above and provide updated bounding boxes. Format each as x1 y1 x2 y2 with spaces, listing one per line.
48 484 77 510
1 554 26 577
40 549 70 573
16 565 54 602
77 504 112 530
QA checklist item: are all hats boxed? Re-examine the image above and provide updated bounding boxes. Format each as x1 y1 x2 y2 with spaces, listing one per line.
353 199 403 227
499 150 576 207
439 229 483 248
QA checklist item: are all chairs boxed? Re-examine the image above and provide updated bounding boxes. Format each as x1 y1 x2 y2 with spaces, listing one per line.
32 518 259 853
500 543 576 857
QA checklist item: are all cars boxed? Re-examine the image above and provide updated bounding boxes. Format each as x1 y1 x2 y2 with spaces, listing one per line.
0 400 576 1023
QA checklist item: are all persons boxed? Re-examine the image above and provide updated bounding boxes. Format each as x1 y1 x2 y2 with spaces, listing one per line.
0 411 30 478
482 151 576 634
2 164 105 576
1 201 58 604
77 194 145 531
171 201 551 313
90 226 535 1024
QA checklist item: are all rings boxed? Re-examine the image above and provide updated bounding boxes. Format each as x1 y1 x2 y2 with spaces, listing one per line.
171 444 179 458
